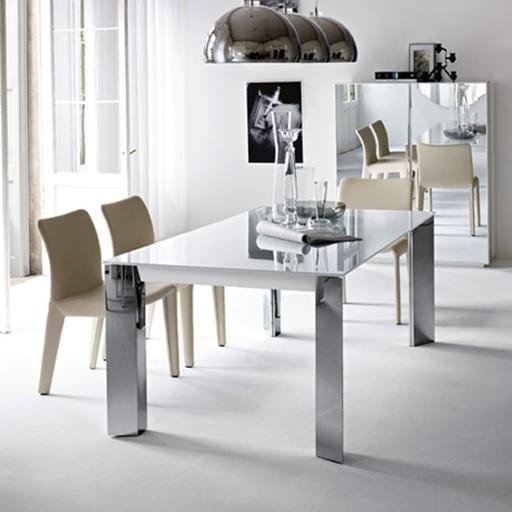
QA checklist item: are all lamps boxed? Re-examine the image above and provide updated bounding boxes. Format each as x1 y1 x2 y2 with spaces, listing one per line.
203 0 300 63
280 0 330 63
309 0 358 62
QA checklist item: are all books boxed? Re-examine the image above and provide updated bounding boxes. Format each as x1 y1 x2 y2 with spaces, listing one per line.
255 220 362 245
256 234 334 255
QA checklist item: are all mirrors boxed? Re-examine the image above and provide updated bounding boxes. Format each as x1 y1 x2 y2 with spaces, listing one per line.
419 82 486 111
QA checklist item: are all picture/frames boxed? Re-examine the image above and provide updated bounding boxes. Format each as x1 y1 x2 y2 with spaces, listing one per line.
408 43 436 79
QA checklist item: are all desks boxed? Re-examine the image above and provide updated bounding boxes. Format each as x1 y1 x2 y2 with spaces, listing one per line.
405 119 488 188
102 207 435 464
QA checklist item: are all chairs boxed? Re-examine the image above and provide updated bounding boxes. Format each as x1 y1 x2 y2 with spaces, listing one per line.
369 119 406 158
89 196 226 369
337 177 415 325
355 126 411 179
415 143 481 236
37 209 179 395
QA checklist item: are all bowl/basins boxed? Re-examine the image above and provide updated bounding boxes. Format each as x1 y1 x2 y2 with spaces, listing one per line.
442 129 477 139
288 200 346 219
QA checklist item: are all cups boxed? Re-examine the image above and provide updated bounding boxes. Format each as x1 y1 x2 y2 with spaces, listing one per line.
271 109 300 225
455 82 473 134
